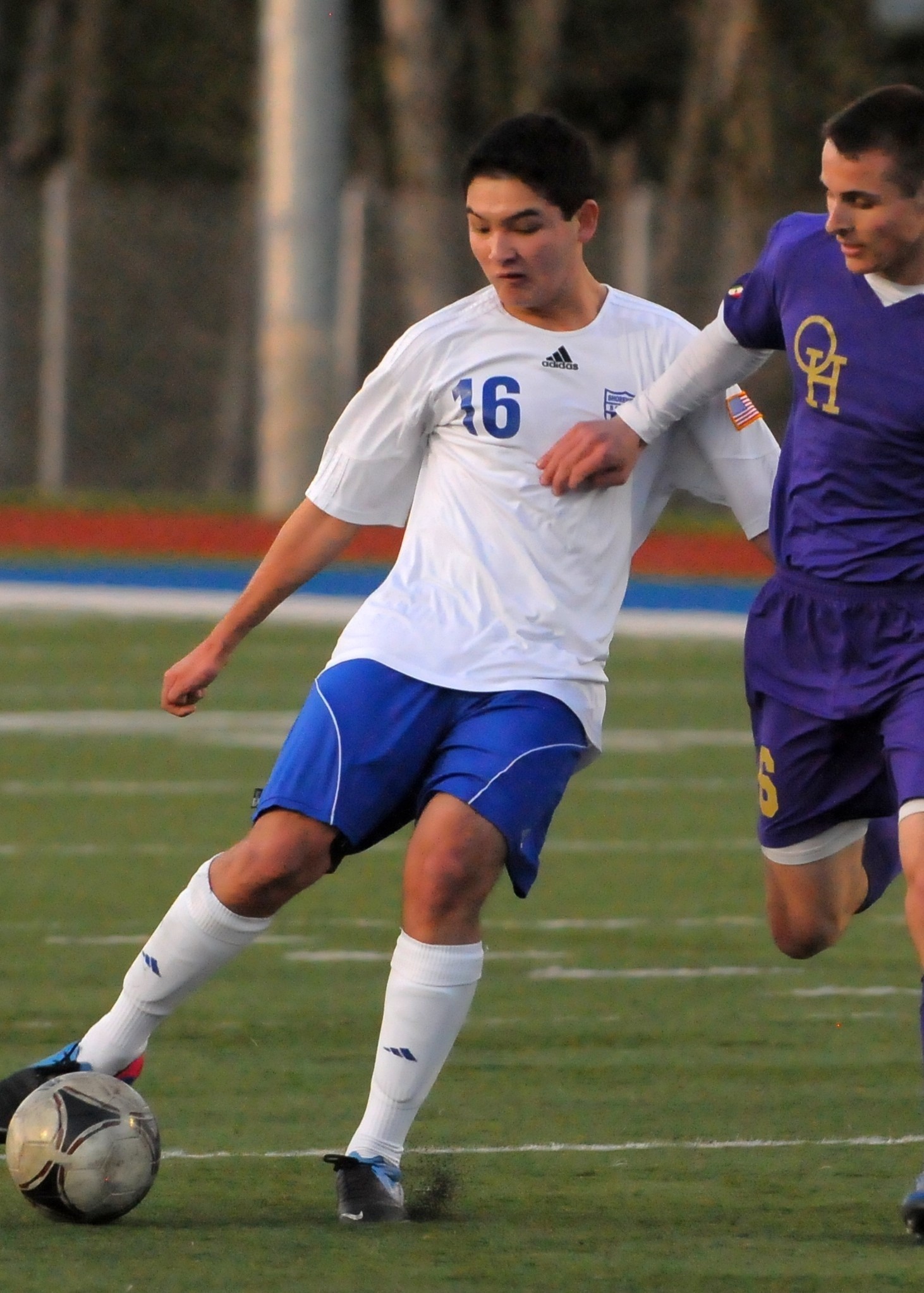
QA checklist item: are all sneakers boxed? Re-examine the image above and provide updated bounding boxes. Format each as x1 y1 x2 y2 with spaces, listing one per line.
322 1150 404 1221
0 1038 144 1145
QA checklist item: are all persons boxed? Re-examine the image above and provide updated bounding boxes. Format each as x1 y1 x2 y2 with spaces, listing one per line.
536 87 924 987
0 112 780 1223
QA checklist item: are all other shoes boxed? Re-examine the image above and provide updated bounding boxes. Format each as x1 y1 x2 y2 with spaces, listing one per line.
900 1173 924 1243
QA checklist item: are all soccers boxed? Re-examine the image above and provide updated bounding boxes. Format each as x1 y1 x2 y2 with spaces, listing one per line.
6 1071 162 1222
